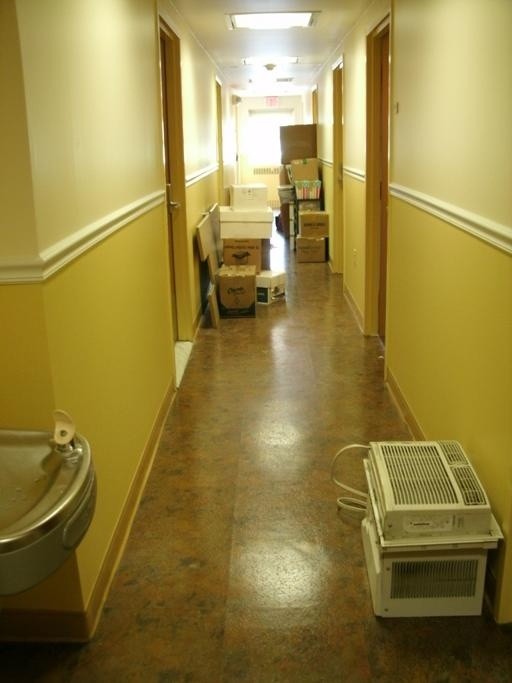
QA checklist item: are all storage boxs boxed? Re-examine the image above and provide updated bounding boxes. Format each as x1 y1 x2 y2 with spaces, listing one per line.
219 183 286 318
276 124 329 263
